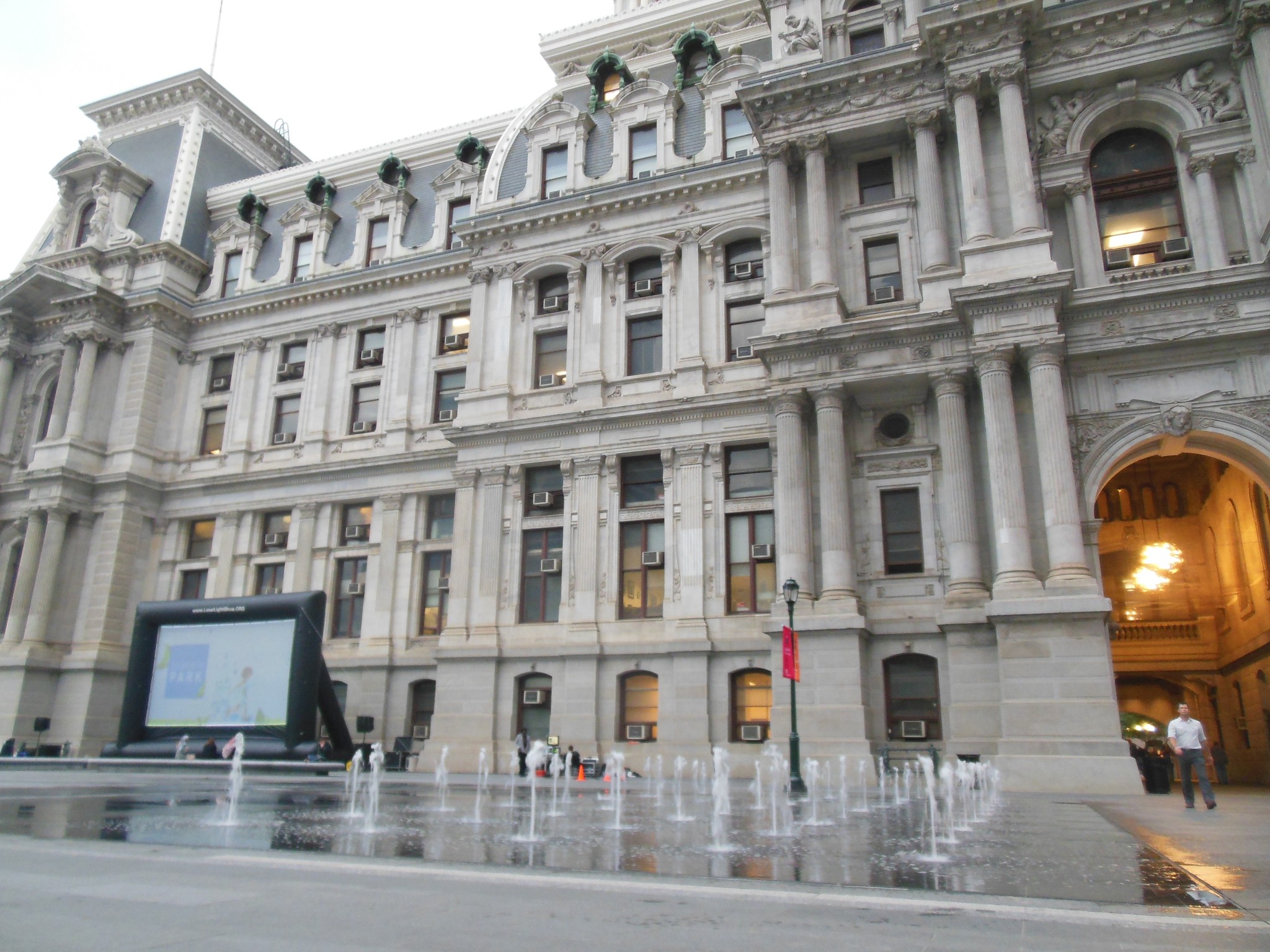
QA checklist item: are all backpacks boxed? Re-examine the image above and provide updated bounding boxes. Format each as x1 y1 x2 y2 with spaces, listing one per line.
572 751 580 763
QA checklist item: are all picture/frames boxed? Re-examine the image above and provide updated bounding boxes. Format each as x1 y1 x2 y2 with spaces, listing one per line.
627 580 635 599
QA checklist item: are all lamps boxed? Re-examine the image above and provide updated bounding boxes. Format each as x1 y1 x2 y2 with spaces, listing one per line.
1129 501 1186 592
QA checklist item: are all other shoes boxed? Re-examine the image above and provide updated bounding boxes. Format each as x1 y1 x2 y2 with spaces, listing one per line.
1207 802 1216 809
1186 804 1194 808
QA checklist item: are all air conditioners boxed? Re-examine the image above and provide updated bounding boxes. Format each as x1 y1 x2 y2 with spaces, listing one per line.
735 345 757 360
641 550 665 566
1158 236 1191 258
293 274 307 283
538 373 560 388
547 188 564 200
438 409 456 421
276 362 296 378
900 717 927 740
733 261 757 279
626 724 651 741
360 348 380 364
635 168 656 180
540 558 561 573
749 539 775 563
634 279 656 297
344 524 363 539
521 690 547 705
264 588 280 594
352 421 373 433
346 581 364 597
740 724 768 742
212 377 229 389
264 533 284 546
413 725 430 739
273 431 294 445
437 576 450 592
542 295 564 313
871 284 898 304
733 148 752 159
1104 246 1132 268
369 258 385 267
450 240 465 249
443 334 465 349
532 490 556 509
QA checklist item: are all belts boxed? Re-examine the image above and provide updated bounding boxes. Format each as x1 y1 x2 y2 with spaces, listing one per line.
1182 749 1199 751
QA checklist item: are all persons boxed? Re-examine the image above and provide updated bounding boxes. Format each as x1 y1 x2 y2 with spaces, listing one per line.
174 733 237 761
313 738 332 778
514 727 531 777
1167 701 1217 810
1210 740 1230 787
0 738 29 758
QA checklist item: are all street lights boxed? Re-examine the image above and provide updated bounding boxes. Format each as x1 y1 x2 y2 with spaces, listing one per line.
782 577 810 796
